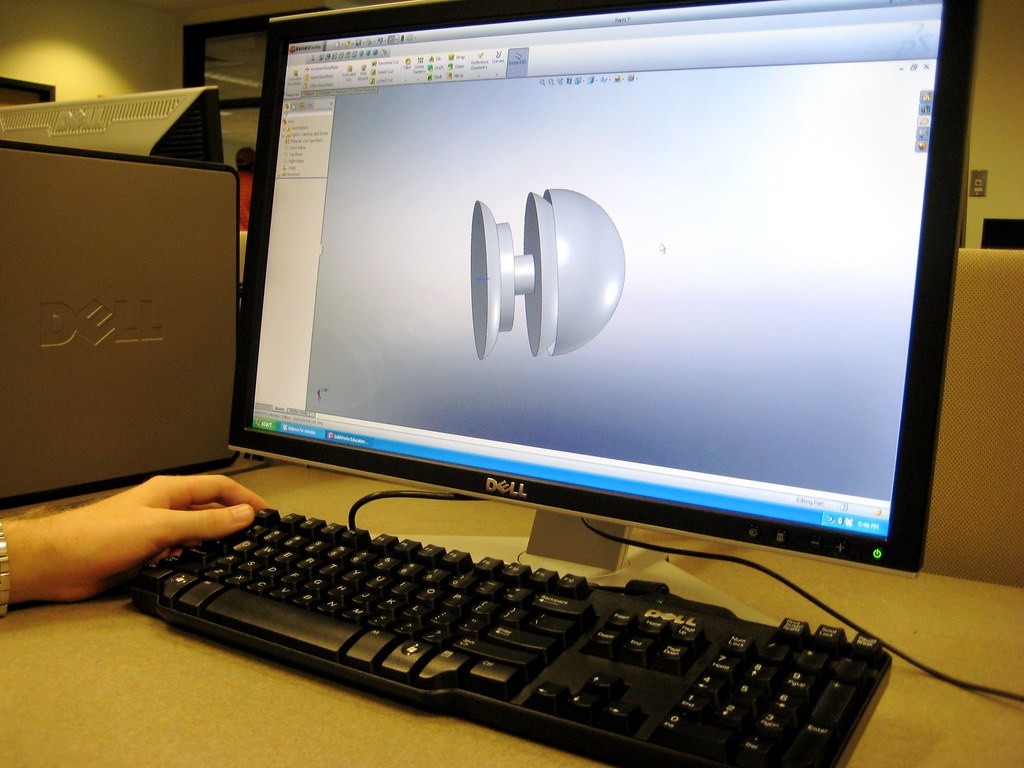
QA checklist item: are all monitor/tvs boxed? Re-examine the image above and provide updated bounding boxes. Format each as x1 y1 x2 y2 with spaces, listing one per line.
228 1 983 630
0 86 224 165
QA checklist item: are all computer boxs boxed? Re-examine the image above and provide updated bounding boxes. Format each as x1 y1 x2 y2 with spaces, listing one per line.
0 140 241 510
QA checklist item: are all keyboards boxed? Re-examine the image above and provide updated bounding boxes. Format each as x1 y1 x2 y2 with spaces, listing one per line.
132 507 893 768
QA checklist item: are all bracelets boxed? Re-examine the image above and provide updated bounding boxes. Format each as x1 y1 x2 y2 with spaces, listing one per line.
0 523 9 617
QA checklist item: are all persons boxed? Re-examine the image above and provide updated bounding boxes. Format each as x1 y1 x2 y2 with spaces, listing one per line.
235 146 258 283
0 474 270 608
